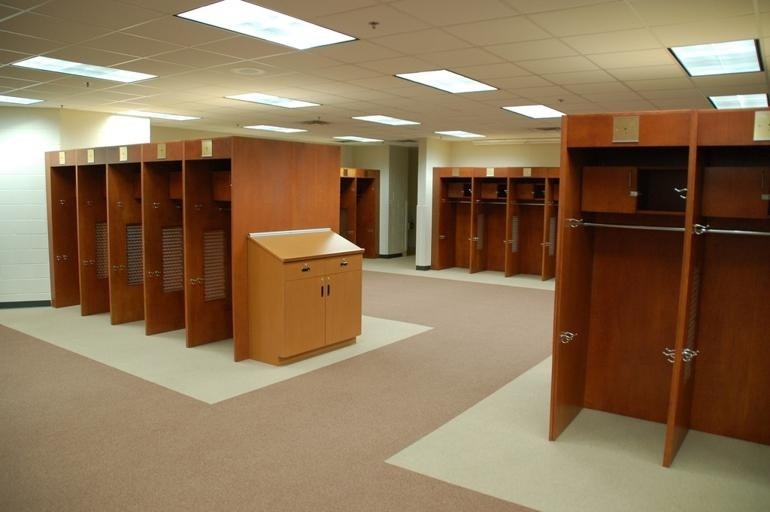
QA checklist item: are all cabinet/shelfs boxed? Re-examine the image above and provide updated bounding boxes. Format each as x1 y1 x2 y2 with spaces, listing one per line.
246 227 366 367
